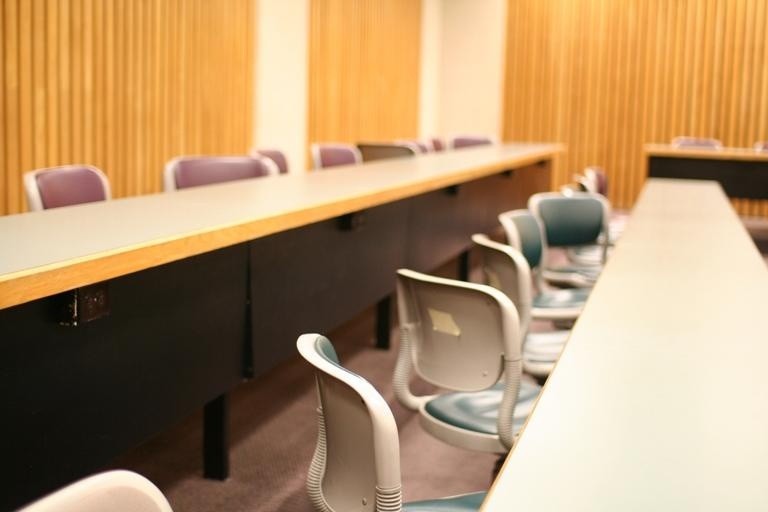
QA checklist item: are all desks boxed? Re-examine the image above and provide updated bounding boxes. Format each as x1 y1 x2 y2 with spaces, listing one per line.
0 143 564 512
477 177 768 511
643 145 768 199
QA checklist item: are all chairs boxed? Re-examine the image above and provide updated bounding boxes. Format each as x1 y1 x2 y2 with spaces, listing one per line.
24 135 493 210
296 164 627 512
